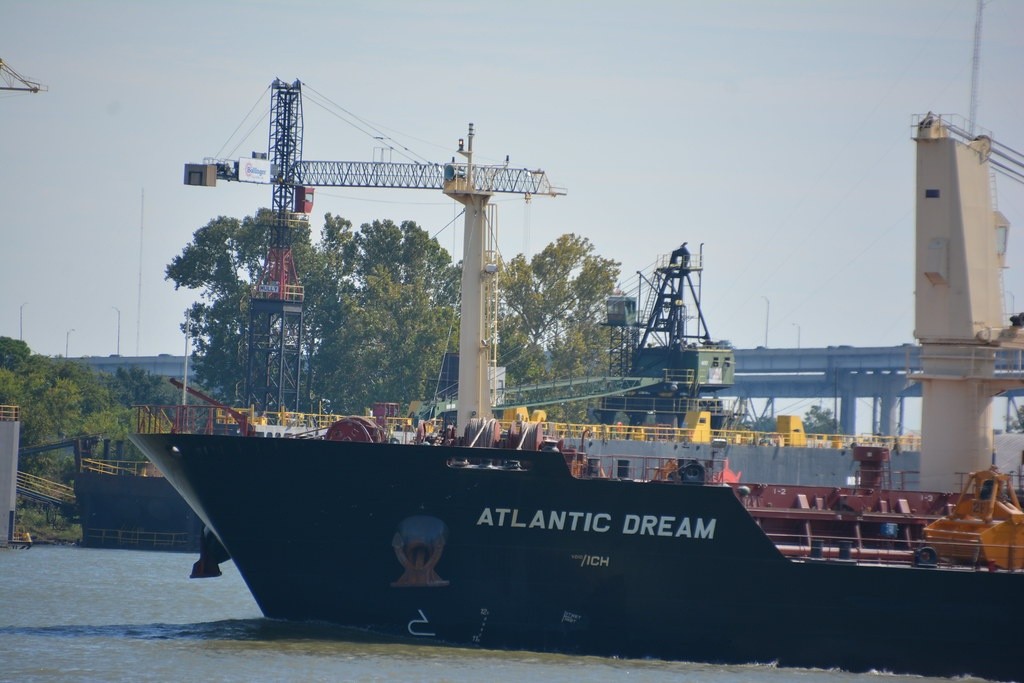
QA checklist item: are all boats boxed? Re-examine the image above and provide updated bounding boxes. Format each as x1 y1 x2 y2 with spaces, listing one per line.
115 77 1024 672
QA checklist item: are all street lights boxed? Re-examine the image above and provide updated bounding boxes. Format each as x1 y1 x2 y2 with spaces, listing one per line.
65 329 76 357
758 294 770 346
791 323 801 350
111 306 121 356
18 301 30 342
